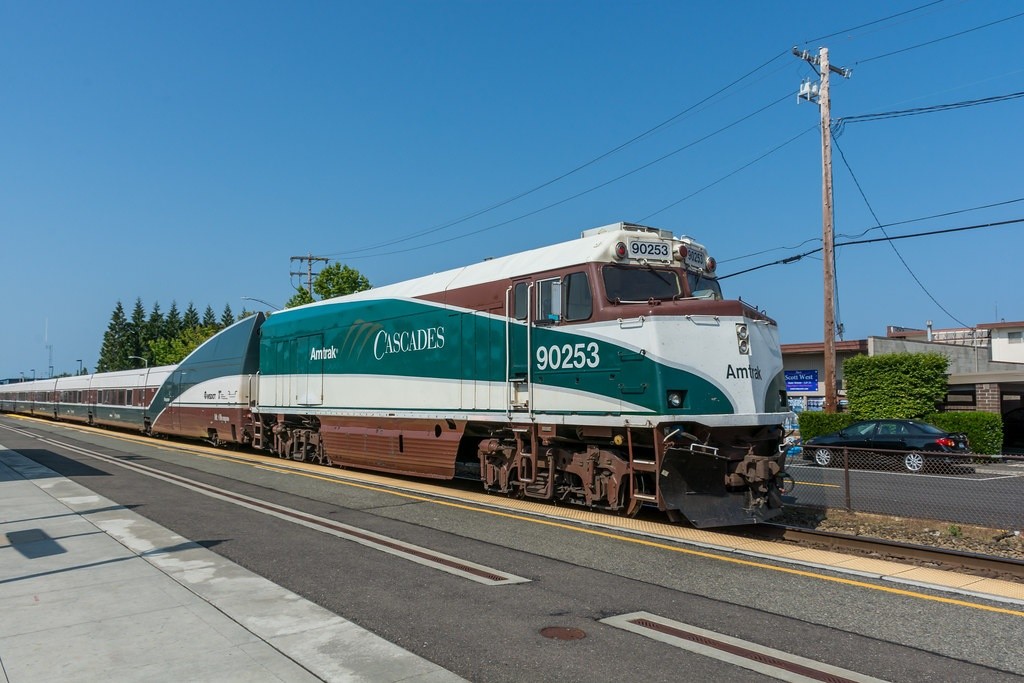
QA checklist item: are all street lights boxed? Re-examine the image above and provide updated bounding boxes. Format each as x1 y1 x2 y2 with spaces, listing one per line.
76 359 82 375
30 370 36 380
128 356 148 369
49 366 53 378
20 371 24 382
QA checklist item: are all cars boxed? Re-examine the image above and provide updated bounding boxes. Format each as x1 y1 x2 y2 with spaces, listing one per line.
804 418 974 476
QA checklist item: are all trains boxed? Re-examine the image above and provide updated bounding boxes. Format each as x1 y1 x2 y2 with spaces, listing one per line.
0 222 823 537
